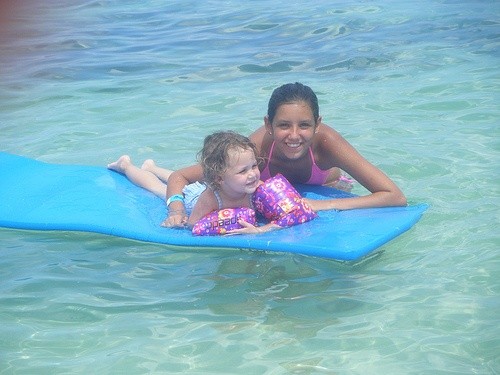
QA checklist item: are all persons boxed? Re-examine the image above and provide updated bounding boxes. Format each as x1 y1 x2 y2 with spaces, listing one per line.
107 129 284 237
160 82 407 228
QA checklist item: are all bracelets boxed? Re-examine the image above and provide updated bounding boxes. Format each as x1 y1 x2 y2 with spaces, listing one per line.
167 193 184 207
